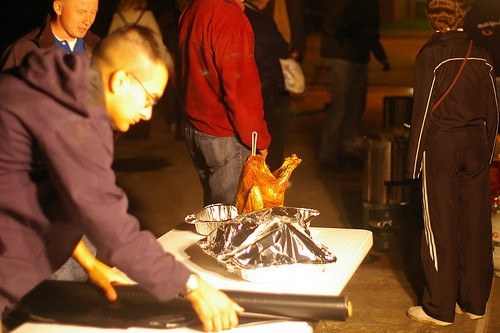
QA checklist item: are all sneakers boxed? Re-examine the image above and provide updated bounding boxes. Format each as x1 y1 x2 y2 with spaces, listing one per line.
407 305 454 327
455 303 488 321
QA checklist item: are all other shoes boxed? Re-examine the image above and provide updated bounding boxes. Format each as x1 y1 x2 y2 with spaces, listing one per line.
321 154 364 170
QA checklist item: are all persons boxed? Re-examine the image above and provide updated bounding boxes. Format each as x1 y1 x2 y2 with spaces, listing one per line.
0 0 103 73
0 25 245 333
318 0 391 170
241 0 306 176
178 0 271 207
407 0 499 326
107 0 176 150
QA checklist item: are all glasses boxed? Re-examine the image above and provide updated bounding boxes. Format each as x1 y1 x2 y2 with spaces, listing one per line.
128 71 158 107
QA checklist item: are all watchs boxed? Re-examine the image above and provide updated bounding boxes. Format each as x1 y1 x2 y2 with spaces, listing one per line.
178 272 200 297
